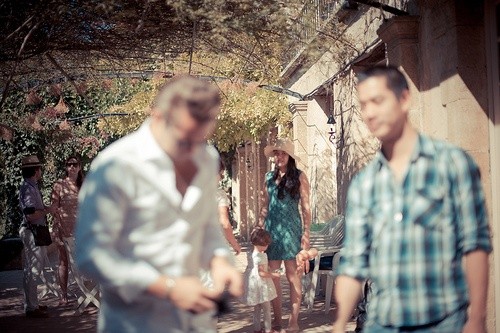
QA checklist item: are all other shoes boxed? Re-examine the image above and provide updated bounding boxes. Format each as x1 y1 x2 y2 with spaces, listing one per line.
25 309 48 318
59 294 69 305
38 304 48 311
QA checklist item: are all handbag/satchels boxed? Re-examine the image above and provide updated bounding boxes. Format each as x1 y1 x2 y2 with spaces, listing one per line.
33 226 52 246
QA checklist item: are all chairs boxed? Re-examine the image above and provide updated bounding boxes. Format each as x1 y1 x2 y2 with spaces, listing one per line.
0 237 35 314
301 214 346 317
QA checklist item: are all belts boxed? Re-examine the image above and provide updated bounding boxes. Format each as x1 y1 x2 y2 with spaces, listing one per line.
384 302 470 331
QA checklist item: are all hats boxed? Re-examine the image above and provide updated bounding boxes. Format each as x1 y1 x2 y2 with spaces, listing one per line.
19 156 42 168
264 139 301 162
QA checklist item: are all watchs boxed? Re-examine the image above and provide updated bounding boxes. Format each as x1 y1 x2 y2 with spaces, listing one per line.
164 277 175 302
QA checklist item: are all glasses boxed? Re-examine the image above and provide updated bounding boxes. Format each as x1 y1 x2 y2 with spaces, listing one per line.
67 163 78 167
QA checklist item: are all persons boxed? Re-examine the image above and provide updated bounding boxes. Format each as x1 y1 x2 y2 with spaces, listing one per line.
244 230 280 333
333 63 493 333
74 73 242 333
216 158 241 313
17 156 57 317
261 138 310 333
52 156 84 304
